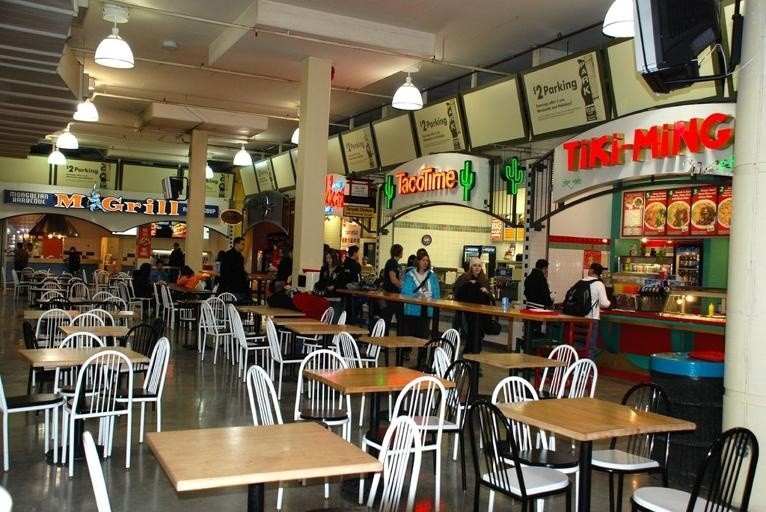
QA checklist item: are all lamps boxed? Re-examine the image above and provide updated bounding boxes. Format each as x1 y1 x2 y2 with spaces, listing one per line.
291 128 298 144
56 122 78 149
47 147 66 164
73 77 99 122
206 162 213 178
93 3 135 68
233 144 253 166
601 0 636 38
392 64 423 110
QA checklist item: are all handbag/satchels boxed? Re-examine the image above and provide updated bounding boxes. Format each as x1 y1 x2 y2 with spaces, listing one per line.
485 320 502 335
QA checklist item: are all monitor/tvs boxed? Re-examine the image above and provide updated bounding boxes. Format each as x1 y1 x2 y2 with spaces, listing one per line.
633 1 722 94
161 176 181 200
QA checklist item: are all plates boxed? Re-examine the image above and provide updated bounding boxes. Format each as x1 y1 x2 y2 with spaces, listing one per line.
643 197 734 230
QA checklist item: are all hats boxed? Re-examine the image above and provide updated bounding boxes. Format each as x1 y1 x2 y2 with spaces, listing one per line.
469 256 482 268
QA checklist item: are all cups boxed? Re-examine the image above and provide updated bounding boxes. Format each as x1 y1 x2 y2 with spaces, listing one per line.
424 291 432 297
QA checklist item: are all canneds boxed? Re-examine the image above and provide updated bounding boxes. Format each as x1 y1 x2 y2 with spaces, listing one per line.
501 297 510 310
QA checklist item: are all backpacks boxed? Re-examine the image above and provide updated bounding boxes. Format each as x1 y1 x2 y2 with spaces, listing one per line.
563 279 603 316
454 280 491 322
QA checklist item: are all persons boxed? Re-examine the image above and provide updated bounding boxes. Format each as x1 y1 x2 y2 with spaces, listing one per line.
581 262 611 360
267 279 302 312
149 259 170 284
168 242 185 267
382 243 405 337
216 238 253 302
177 264 210 289
277 247 295 288
453 257 495 353
69 247 80 277
132 262 163 317
12 242 28 282
401 247 440 339
318 244 364 324
524 257 557 311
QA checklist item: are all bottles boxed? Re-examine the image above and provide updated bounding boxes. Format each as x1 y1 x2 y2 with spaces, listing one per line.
640 284 667 307
678 253 699 285
